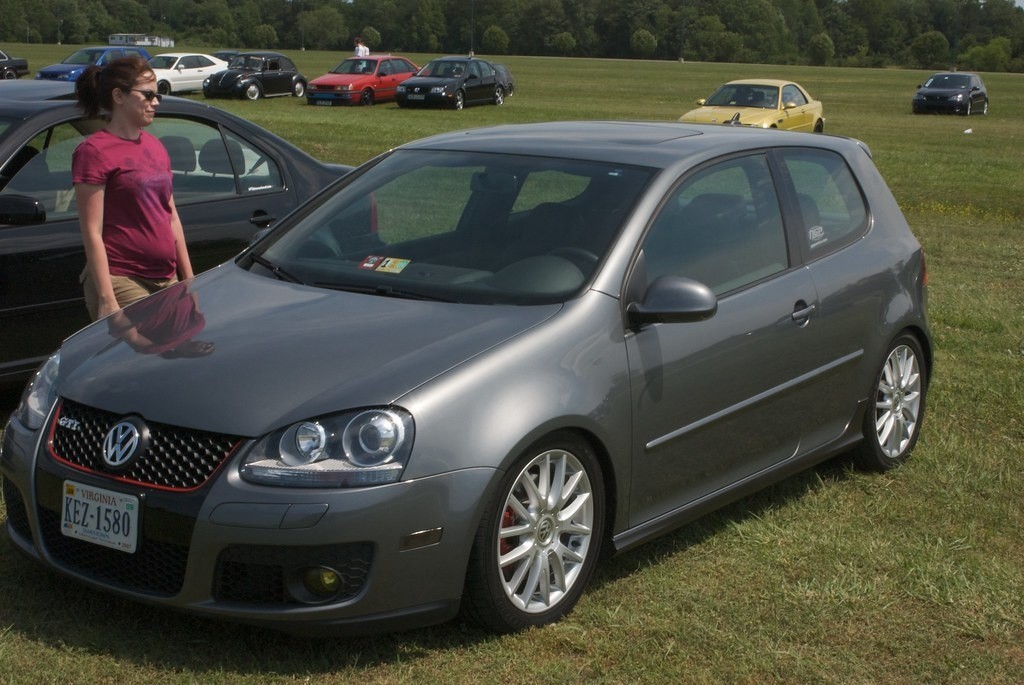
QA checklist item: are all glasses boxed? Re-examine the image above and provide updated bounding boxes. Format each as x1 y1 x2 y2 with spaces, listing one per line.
202 343 213 352
132 89 162 103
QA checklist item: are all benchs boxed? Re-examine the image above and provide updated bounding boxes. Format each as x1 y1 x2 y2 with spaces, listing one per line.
598 185 819 270
156 136 245 194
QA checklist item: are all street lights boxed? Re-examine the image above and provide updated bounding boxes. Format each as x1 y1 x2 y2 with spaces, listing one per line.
160 15 166 47
57 18 64 44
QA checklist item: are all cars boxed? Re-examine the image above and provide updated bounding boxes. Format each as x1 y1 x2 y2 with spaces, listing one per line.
0 122 934 635
36 46 152 82
395 55 514 110
912 72 989 117
678 79 825 133
202 51 307 100
147 53 229 95
0 49 30 80
305 55 430 106
0 79 386 429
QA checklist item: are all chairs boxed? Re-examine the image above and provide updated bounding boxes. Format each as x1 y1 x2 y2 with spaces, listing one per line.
471 202 582 267
748 90 768 105
0 146 49 198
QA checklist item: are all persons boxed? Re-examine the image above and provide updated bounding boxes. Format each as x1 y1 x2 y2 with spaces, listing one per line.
71 56 196 323
355 41 369 56
354 37 364 58
107 282 215 359
